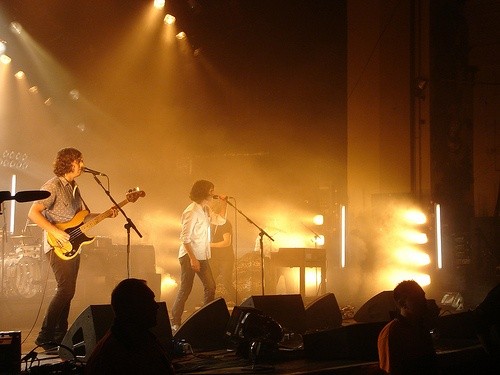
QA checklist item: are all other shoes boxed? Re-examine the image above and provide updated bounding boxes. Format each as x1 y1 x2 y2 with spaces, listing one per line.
35 338 59 355
171 324 180 331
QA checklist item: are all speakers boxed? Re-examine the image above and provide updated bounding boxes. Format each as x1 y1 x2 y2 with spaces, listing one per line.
353 290 399 324
242 294 307 339
173 298 231 350
57 303 172 361
304 292 342 331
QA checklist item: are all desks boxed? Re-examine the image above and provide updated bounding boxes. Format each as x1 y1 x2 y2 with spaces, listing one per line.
270 248 326 301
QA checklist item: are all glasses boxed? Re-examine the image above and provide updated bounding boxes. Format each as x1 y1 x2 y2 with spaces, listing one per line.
71 159 83 164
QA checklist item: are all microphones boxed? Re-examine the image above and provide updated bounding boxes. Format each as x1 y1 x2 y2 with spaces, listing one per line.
81 167 106 176
212 195 226 201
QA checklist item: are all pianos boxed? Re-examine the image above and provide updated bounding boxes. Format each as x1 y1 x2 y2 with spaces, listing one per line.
263 247 326 297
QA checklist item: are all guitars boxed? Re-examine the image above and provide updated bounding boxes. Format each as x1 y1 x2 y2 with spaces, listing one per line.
211 224 218 260
47 186 145 260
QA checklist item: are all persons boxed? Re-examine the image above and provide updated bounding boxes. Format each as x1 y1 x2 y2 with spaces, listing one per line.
378 281 440 375
446 286 500 375
171 180 228 332
195 204 243 310
84 277 177 375
26 147 119 352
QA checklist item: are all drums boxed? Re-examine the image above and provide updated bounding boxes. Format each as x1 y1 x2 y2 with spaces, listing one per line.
0 244 46 299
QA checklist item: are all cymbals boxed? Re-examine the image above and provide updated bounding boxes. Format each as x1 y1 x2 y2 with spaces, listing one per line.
27 224 39 227
10 235 33 239
